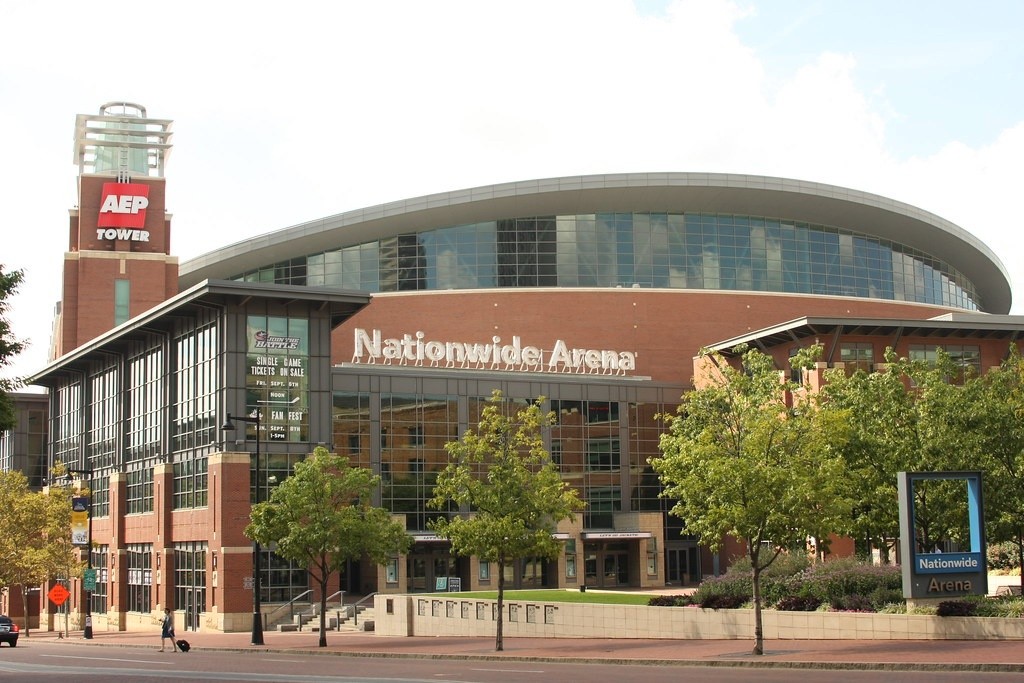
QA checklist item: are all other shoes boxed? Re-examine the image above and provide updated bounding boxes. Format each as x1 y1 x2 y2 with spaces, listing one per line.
171 651 177 653
157 650 164 652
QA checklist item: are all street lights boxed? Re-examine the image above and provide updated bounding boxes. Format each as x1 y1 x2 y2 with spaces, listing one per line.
62 462 93 638
219 406 267 646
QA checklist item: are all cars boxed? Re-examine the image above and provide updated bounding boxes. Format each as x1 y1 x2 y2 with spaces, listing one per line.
0 615 20 647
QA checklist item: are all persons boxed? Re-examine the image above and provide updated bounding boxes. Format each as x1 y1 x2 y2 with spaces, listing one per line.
157 607 177 652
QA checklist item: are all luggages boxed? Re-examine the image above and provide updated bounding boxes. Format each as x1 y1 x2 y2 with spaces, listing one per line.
168 631 190 652
